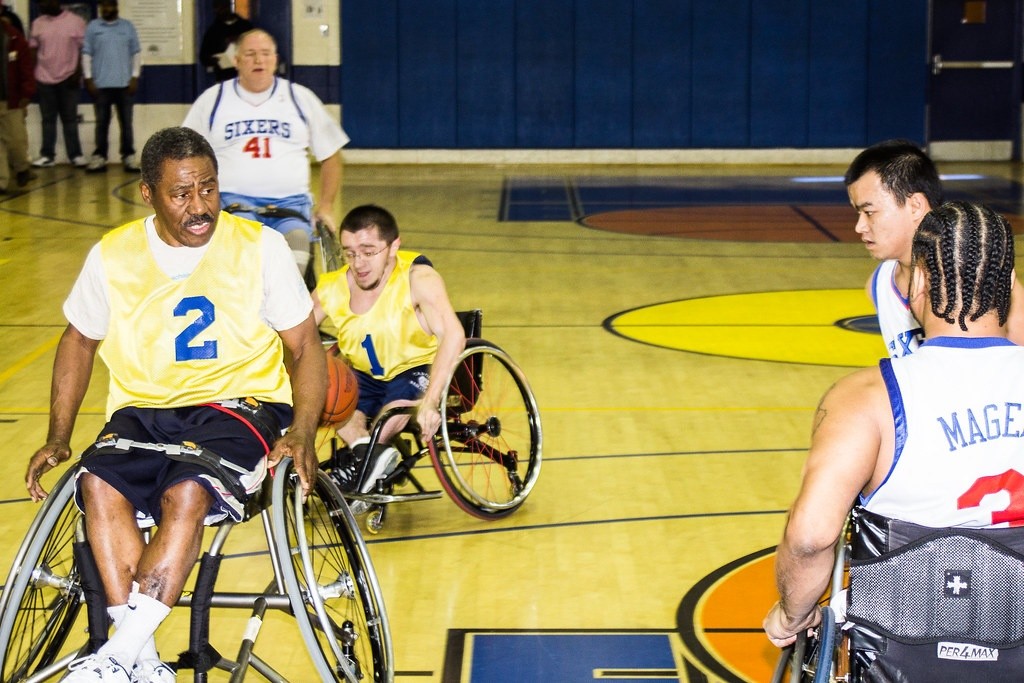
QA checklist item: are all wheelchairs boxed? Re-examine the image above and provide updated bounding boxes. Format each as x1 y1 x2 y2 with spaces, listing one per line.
772 498 1024 683
310 309 544 535
219 203 346 272
0 427 394 683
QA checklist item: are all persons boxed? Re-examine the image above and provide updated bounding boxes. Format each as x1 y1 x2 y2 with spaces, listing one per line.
27 0 88 169
81 0 142 174
0 0 34 194
845 139 1024 359
309 204 466 508
762 199 1024 647
25 127 328 683
180 28 350 281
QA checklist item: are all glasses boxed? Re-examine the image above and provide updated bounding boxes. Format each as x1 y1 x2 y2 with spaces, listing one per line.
343 244 392 261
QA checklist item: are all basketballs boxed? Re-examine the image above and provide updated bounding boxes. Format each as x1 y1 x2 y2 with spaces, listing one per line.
317 353 359 429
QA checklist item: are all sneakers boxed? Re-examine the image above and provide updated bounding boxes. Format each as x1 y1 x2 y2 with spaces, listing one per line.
350 443 399 515
87 153 106 171
326 459 361 494
32 156 55 168
73 156 88 166
62 654 131 683
123 155 140 171
128 660 176 683
